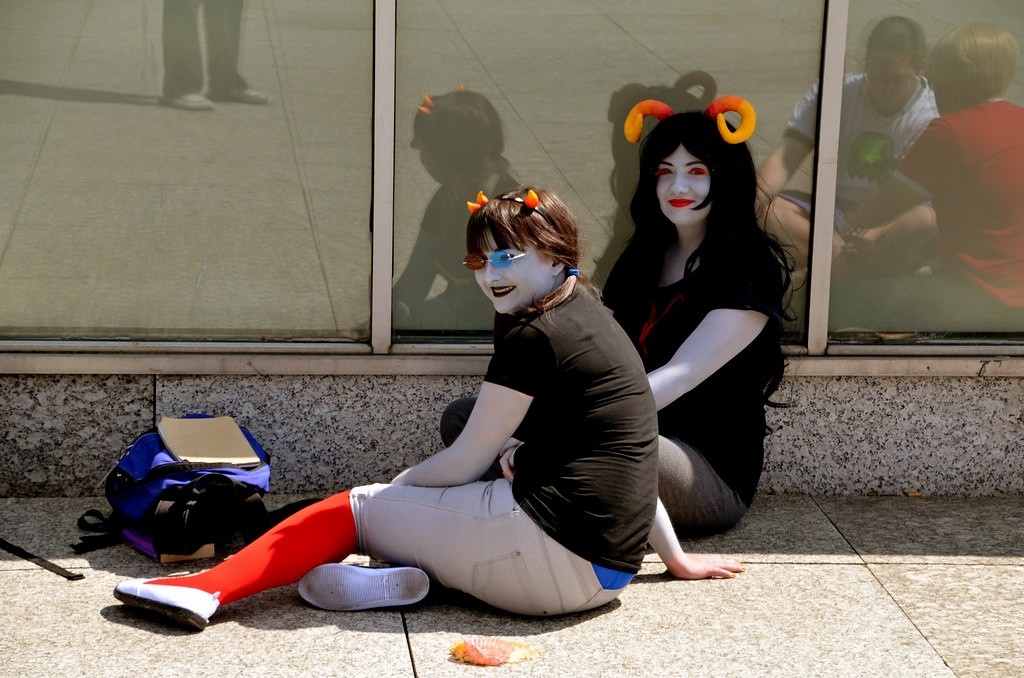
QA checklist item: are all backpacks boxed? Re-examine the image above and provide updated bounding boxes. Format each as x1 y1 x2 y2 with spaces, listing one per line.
69 413 322 563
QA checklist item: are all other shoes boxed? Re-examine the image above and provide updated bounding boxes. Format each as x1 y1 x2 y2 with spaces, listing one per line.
160 96 213 110
114 577 219 631
240 90 270 104
297 563 430 611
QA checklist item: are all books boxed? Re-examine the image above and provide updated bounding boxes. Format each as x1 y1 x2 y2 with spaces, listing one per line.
154 413 261 466
124 530 216 563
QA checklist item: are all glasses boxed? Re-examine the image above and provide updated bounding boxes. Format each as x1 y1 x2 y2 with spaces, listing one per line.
462 248 534 270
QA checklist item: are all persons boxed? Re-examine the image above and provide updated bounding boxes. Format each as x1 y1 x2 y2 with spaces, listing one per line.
159 0 271 107
356 16 1024 329
113 185 746 632
441 95 791 539
